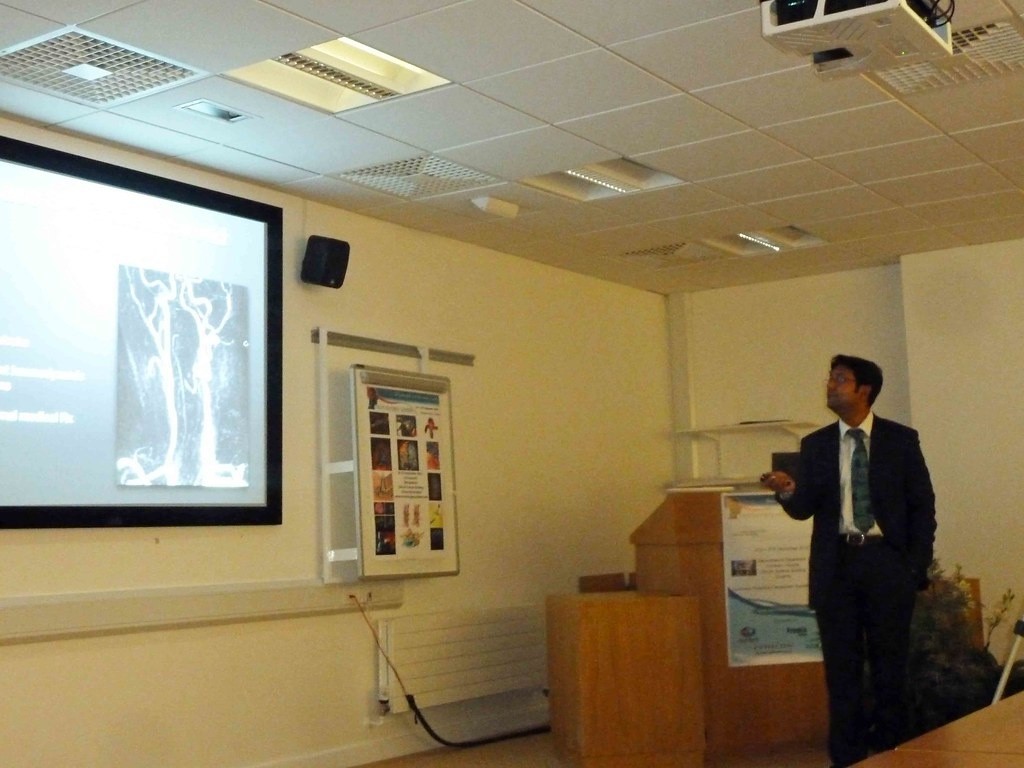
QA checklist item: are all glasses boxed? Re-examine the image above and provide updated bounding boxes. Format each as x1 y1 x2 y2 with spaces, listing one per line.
824 376 856 384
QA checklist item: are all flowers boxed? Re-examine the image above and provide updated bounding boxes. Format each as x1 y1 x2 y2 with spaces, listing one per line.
907 562 1014 728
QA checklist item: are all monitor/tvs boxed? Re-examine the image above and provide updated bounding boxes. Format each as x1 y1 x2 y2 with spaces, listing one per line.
771 453 800 481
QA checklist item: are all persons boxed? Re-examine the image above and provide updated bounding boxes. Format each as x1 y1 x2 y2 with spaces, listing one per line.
758 355 938 768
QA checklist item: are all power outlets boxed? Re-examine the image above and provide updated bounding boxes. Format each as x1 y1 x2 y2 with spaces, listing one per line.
343 587 360 604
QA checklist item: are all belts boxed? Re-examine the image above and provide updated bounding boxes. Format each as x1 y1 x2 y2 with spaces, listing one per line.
837 533 884 545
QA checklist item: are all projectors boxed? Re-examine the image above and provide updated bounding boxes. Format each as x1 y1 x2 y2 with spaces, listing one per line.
759 0 957 82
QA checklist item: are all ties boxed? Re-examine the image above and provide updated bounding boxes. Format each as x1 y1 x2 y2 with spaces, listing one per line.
847 429 874 534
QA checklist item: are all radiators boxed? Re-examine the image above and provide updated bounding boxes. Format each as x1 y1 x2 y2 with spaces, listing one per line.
377 606 547 713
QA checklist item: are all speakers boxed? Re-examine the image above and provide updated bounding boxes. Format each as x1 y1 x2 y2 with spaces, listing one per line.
300 236 349 289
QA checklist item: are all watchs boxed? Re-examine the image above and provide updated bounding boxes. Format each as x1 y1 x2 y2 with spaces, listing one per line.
780 492 794 500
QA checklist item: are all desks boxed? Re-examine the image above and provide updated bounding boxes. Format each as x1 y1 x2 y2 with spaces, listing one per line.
545 593 705 768
850 690 1024 768
